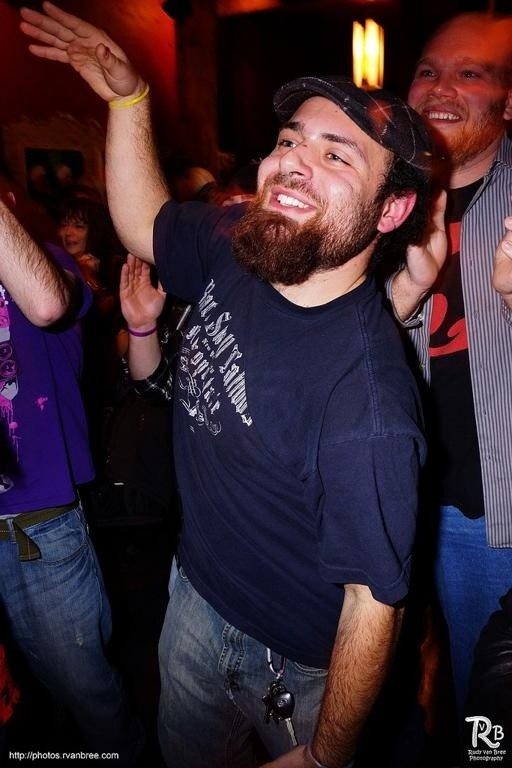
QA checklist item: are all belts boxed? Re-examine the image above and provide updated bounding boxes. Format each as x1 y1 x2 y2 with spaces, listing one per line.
0 500 79 561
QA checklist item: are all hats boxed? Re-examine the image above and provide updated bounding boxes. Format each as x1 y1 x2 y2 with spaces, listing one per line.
272 76 434 171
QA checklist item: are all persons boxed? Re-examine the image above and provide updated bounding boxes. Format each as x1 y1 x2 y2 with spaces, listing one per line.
2 1 511 768
